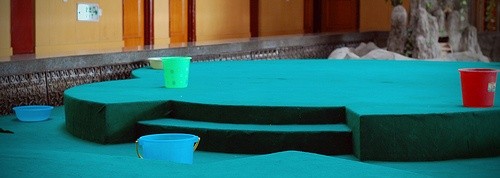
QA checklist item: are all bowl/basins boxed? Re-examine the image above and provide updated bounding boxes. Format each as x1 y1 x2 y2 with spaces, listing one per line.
10 106 55 122
147 57 163 68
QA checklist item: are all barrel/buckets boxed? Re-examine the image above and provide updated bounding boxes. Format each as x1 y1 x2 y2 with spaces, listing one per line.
161 54 192 90
458 66 500 110
134 132 200 163
